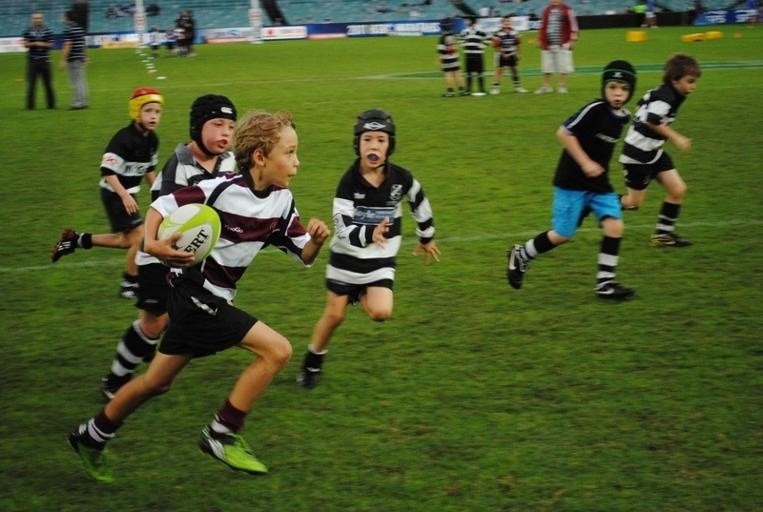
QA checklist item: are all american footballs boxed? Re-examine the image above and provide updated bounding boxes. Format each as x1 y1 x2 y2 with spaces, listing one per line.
156 203 221 266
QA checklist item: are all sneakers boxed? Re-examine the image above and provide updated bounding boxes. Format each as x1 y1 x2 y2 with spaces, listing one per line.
51 230 77 262
508 244 528 290
198 424 269 476
594 279 636 299
67 425 116 483
302 347 327 388
649 234 690 248
100 373 132 401
443 85 568 96
118 277 138 301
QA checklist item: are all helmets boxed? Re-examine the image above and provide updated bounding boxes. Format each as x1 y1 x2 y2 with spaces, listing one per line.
190 95 238 142
353 110 396 155
601 61 637 104
129 87 163 120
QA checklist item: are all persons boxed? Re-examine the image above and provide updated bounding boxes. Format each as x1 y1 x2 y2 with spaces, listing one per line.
52 89 161 296
66 110 331 485
293 109 441 391
148 9 195 56
23 11 55 110
459 17 488 93
491 14 527 92
100 95 235 400
535 0 578 93
59 10 92 111
436 19 462 95
616 53 699 249
506 60 634 299
